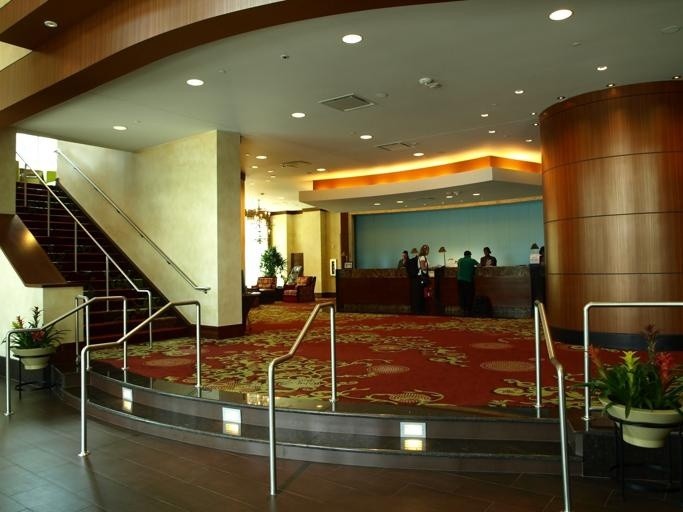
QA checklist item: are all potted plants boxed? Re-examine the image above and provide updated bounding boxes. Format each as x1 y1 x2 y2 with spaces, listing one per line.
257 247 286 291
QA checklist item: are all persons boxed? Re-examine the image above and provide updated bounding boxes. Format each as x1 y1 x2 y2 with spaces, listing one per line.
539 246 545 263
480 247 497 266
411 244 430 315
456 251 479 312
397 250 411 268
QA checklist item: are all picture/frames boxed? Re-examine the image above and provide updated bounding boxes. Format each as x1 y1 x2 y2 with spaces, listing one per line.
344 262 353 269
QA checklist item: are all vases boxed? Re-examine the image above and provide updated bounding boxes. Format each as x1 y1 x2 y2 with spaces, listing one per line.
598 392 683 449
10 345 55 370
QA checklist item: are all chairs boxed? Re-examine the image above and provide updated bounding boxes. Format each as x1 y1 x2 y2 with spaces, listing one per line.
16 161 57 186
283 276 316 303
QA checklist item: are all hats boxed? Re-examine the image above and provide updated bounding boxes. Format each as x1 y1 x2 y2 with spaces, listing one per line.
464 251 472 256
484 247 491 254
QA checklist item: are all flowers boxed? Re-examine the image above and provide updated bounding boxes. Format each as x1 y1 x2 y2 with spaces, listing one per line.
570 324 683 420
0 306 72 352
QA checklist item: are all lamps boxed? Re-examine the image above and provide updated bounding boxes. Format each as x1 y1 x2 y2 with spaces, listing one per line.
244 196 271 244
438 246 447 268
410 248 418 257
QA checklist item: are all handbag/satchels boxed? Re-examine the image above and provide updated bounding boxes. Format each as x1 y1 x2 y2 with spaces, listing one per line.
420 266 429 285
475 293 494 318
